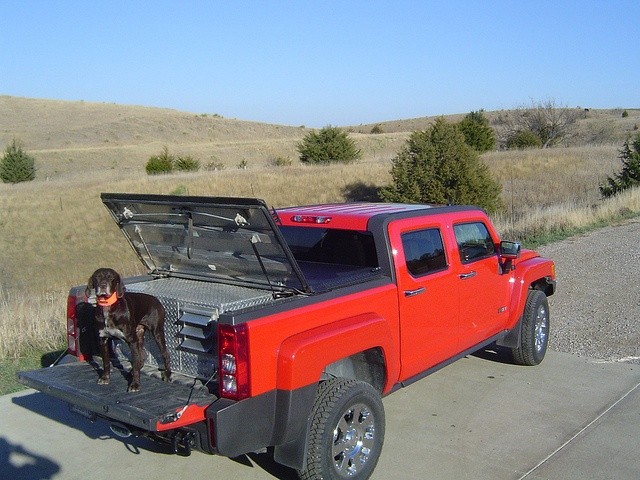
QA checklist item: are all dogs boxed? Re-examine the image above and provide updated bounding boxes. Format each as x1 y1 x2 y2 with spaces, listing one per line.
85 268 172 392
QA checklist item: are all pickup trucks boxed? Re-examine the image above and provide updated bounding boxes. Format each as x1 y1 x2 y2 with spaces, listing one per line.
15 192 557 479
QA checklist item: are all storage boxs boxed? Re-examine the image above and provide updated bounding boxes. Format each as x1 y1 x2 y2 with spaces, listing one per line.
111 279 281 383
